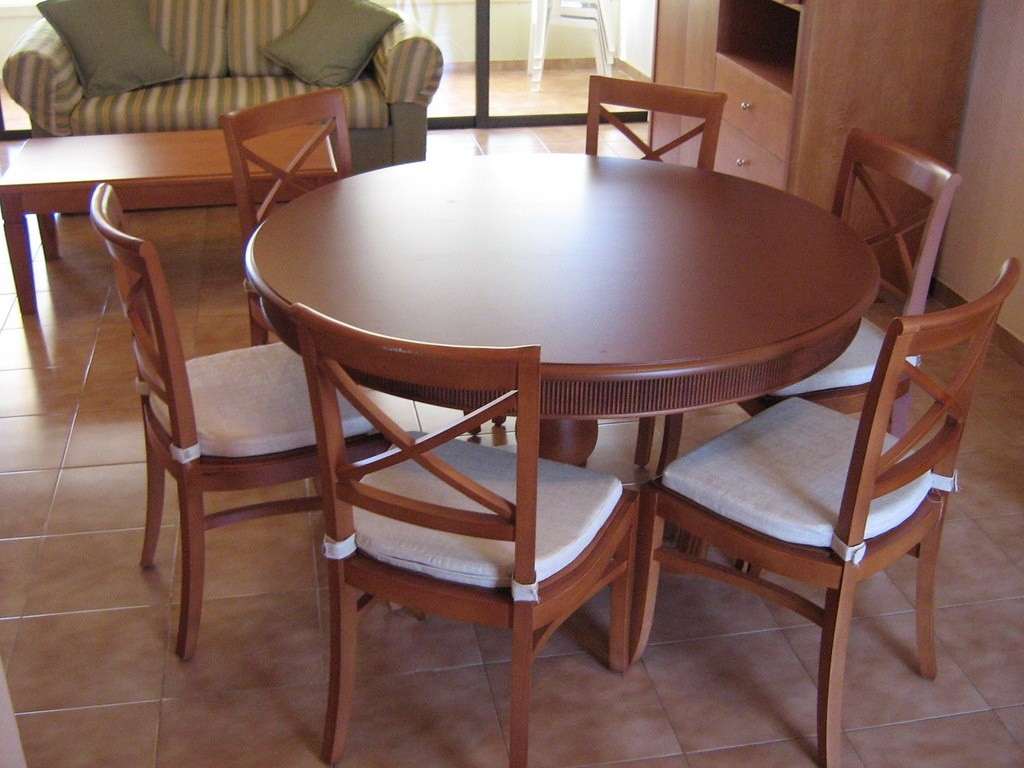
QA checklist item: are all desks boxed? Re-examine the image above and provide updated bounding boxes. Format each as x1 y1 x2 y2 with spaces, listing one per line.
0 124 348 317
243 152 881 619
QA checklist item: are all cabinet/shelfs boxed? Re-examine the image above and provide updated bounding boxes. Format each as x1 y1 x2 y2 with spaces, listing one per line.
647 0 983 303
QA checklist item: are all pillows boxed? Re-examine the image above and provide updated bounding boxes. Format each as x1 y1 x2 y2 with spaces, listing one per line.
36 0 187 100
264 0 404 92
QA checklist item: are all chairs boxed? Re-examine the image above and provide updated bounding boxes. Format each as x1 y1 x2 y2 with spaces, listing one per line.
526 0 615 92
88 73 1021 768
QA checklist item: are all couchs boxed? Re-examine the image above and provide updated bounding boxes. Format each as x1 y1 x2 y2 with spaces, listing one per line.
2 0 445 178
